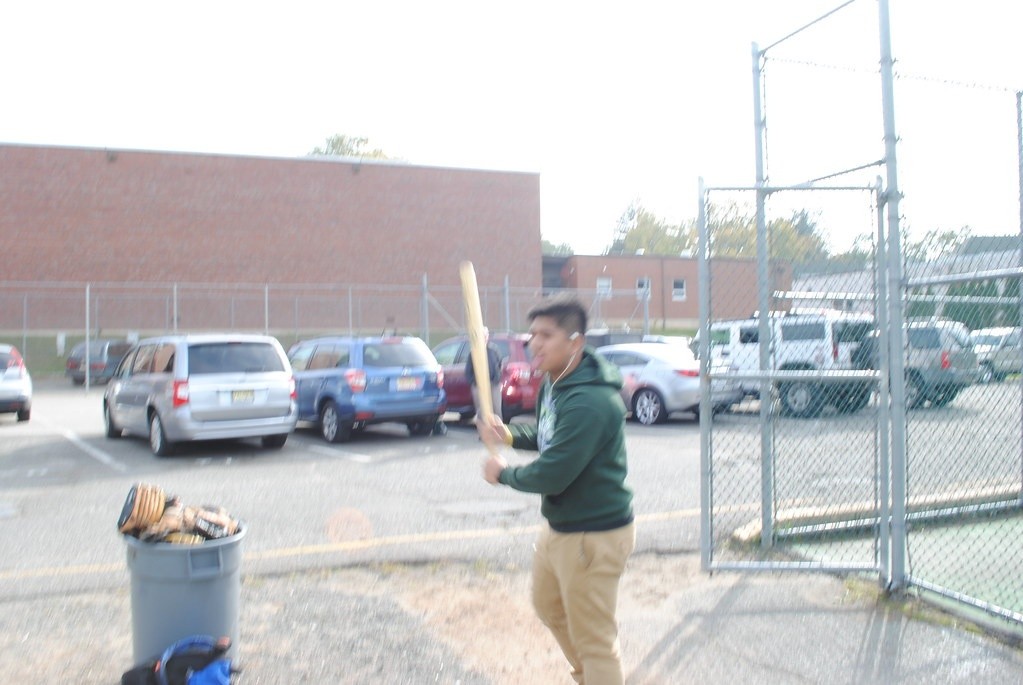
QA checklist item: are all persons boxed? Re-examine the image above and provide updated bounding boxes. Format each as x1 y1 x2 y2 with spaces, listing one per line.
474 301 636 684
466 326 503 440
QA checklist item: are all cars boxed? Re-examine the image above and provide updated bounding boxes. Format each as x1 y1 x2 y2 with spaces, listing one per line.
67 338 127 386
592 342 745 424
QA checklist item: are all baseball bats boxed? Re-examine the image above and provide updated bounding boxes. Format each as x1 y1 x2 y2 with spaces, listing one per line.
458 261 503 461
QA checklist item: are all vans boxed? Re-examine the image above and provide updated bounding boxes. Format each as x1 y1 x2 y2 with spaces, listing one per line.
971 326 1023 382
103 335 300 456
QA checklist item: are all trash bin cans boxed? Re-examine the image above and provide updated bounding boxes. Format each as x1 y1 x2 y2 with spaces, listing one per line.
118 481 240 685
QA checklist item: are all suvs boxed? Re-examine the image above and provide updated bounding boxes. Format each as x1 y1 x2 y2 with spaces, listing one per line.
431 333 544 424
866 317 980 410
285 337 448 444
690 314 873 419
0 344 33 421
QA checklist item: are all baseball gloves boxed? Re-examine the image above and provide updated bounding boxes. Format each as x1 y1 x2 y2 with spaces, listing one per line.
117 481 239 544
